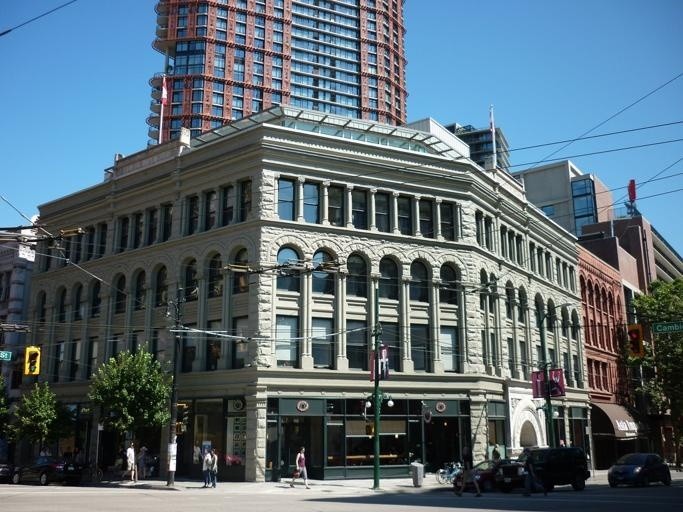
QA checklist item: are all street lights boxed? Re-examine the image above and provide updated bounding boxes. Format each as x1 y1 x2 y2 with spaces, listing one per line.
530 301 577 447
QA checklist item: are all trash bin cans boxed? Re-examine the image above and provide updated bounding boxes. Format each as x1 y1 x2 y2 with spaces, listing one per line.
266 468 273 482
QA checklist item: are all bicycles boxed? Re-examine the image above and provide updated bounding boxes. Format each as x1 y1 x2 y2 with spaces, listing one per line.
435 461 462 485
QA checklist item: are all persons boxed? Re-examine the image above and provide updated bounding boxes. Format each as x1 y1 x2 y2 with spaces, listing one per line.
202 448 212 488
206 448 218 488
40 446 84 481
290 447 311 489
119 441 148 481
523 448 547 496
193 440 203 464
492 444 501 462
455 447 480 497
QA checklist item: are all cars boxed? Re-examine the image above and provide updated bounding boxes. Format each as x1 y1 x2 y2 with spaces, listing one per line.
9 455 81 486
454 459 526 497
606 452 672 487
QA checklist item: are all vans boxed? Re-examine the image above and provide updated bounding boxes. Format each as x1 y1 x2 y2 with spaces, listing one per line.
516 448 590 493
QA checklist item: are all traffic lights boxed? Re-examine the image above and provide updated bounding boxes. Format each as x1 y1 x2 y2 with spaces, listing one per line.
626 323 645 359
24 344 42 376
